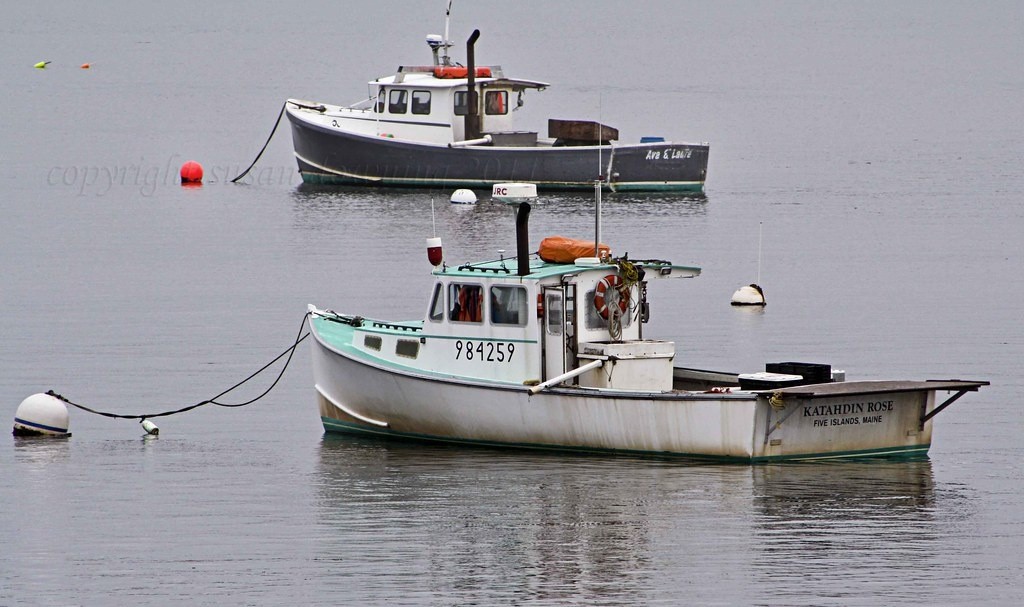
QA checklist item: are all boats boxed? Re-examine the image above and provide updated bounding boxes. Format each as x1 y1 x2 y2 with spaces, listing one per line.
282 1 712 201
304 176 991 466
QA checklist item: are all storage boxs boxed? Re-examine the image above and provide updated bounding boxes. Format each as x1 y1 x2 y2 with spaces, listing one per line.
578 338 674 391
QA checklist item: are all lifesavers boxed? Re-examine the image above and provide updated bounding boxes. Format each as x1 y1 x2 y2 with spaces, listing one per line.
593 275 630 319
